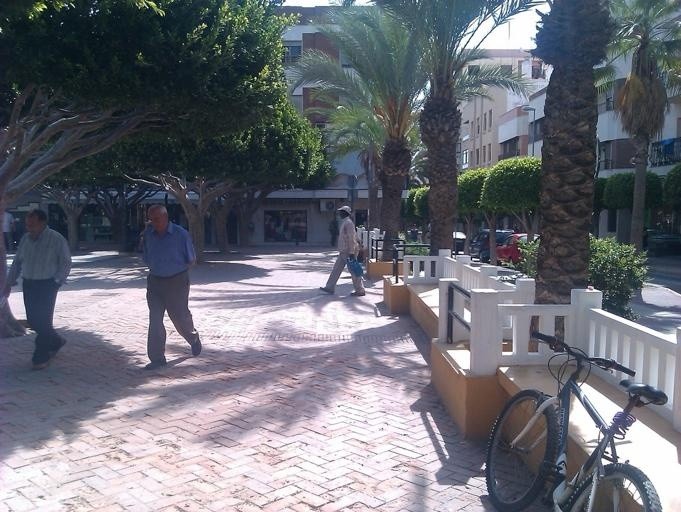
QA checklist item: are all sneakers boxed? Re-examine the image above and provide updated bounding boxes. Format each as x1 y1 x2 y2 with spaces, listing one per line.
48 337 66 357
33 362 48 369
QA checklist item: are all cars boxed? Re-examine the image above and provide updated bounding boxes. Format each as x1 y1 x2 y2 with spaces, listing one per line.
494 233 540 267
452 232 466 253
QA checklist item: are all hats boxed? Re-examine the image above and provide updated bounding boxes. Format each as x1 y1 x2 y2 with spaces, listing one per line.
337 206 352 214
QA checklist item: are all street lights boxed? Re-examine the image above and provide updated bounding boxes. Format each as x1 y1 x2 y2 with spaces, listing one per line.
521 104 536 158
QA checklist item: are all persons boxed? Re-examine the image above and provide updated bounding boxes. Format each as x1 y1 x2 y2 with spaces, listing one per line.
1 206 17 255
2 209 72 371
319 204 367 297
411 223 419 241
138 204 202 369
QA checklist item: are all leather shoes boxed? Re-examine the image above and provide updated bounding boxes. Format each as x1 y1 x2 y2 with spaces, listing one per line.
320 287 335 294
192 340 201 355
146 356 166 369
351 291 366 295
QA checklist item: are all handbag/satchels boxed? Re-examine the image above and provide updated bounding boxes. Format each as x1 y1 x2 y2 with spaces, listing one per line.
346 257 363 276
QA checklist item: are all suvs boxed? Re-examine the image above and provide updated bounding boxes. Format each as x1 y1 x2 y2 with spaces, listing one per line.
469 229 515 263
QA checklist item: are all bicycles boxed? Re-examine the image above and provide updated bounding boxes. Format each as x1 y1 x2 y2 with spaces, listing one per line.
485 331 667 512
